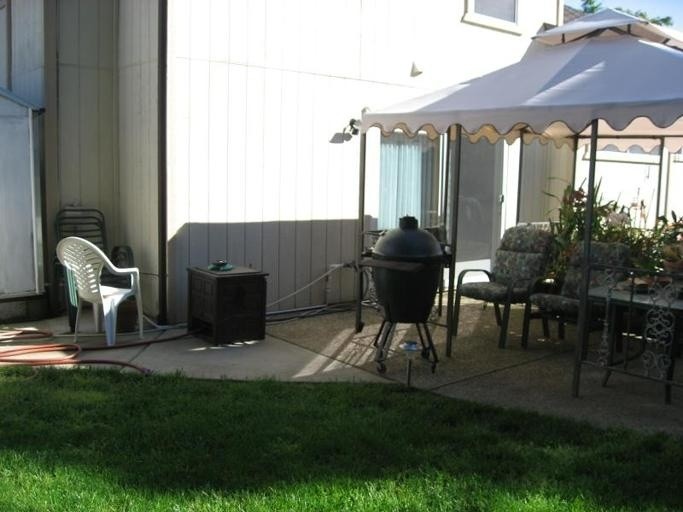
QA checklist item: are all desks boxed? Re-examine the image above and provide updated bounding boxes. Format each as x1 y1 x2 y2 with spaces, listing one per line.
570 271 683 406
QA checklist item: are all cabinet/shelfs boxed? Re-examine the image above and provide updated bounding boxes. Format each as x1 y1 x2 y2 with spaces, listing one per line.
185 259 269 343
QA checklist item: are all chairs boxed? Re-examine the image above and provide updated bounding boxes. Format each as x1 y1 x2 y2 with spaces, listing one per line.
452 222 555 350
521 240 631 362
54 236 144 346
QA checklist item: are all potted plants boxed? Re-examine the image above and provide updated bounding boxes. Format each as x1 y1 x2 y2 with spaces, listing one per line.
652 212 683 270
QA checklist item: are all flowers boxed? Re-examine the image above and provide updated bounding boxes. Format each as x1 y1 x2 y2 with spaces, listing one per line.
605 210 634 242
558 190 585 206
